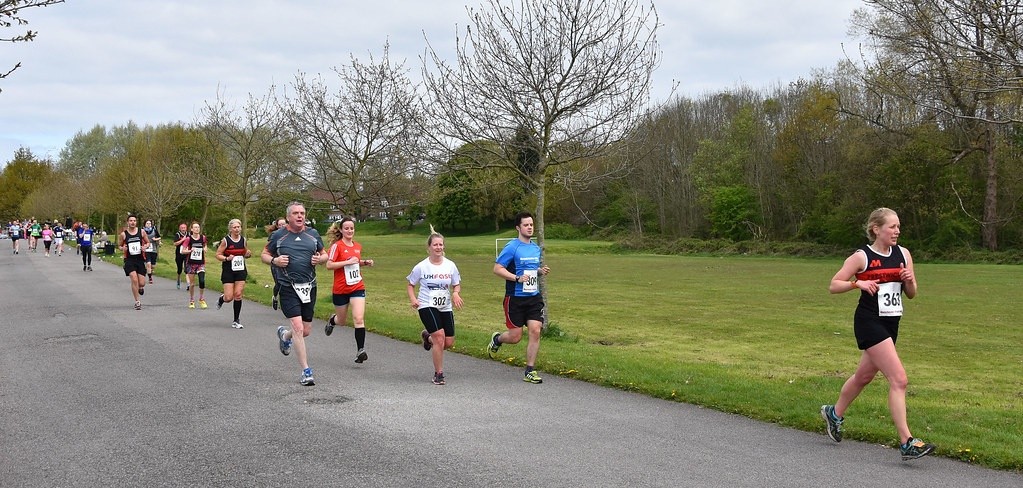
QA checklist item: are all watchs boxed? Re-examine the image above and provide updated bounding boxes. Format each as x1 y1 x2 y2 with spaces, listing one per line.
515 275 520 283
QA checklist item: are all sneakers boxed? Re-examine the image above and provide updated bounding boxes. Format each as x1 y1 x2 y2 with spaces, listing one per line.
421 329 433 351
177 280 180 289
188 300 196 309
186 282 191 290
820 404 844 443
432 371 445 385
299 367 315 386
87 266 93 271
149 276 152 284
83 266 86 271
232 319 244 328
198 298 208 308
133 300 141 310
487 332 502 359
271 295 278 311
355 348 368 363
138 285 145 295
215 293 223 310
897 437 934 461
277 326 293 355
523 369 542 384
324 313 337 336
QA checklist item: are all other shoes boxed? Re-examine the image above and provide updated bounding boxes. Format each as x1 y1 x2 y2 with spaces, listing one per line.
14 249 18 254
58 252 61 256
45 252 50 258
54 250 57 254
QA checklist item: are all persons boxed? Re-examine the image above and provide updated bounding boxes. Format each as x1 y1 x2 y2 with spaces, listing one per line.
487 213 551 384
118 215 150 310
264 218 289 311
142 219 161 284
72 221 92 271
261 202 328 386
182 221 208 308
216 219 251 328
173 224 190 290
406 223 464 385
0 217 64 257
92 231 108 254
821 208 936 461
325 216 373 363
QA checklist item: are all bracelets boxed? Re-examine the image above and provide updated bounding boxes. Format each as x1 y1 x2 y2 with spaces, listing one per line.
851 278 858 290
453 291 460 295
271 258 274 265
225 256 227 262
364 260 367 265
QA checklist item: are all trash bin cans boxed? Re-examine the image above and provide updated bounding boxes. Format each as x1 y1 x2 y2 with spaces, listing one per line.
104 242 117 255
